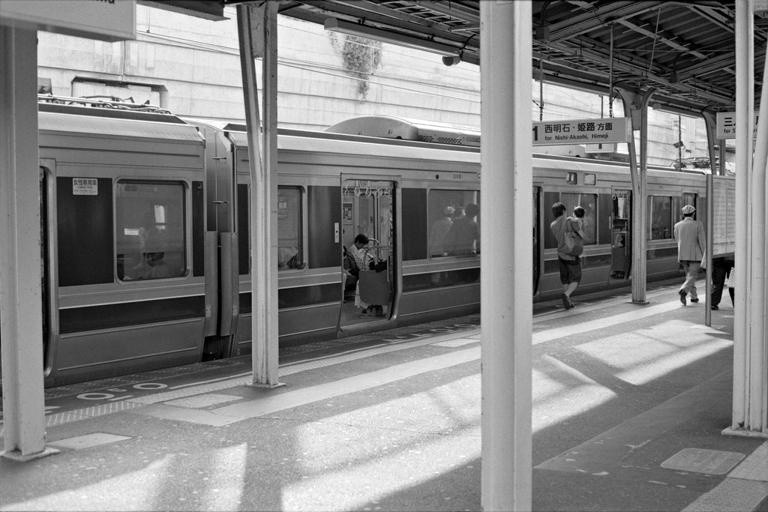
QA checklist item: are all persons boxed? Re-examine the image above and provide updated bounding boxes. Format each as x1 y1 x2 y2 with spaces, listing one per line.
348 234 373 271
566 205 584 262
549 201 582 310
711 258 733 311
356 258 390 316
673 205 704 304
138 212 161 253
430 201 480 284
135 252 180 279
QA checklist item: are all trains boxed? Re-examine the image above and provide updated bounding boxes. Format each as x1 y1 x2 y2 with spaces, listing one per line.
37 78 709 389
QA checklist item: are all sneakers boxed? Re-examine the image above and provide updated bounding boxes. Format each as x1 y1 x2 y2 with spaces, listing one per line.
561 292 575 311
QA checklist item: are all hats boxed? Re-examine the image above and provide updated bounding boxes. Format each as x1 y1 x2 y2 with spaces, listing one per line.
682 205 695 214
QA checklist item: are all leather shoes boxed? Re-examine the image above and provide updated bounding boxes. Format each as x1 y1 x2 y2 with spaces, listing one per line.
691 298 698 302
679 290 687 304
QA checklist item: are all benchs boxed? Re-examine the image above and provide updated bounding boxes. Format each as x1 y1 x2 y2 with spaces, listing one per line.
358 238 393 316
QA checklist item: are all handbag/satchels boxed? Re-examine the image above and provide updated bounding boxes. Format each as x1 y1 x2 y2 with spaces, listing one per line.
564 232 583 256
